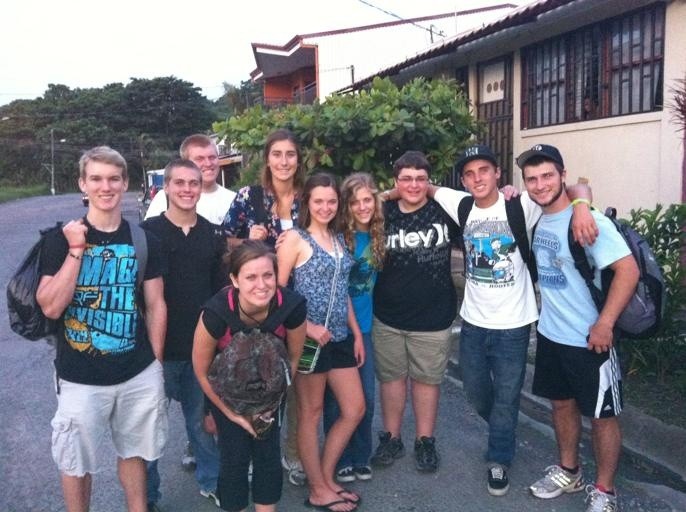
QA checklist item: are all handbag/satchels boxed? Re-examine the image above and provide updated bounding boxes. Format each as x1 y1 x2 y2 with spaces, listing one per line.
292 331 325 375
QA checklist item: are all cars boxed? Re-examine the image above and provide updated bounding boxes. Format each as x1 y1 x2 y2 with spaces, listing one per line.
135 183 162 226
82 192 88 207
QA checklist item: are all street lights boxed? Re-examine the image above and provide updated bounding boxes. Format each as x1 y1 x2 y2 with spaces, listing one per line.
40 129 66 195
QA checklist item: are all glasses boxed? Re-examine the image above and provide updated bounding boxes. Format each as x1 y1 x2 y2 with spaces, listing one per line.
399 176 431 187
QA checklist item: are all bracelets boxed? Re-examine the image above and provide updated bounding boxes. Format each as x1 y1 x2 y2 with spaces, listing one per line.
571 196 592 207
68 243 85 249
384 190 391 202
67 252 82 260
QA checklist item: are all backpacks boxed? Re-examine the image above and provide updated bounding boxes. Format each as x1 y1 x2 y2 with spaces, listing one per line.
199 295 304 413
599 206 666 339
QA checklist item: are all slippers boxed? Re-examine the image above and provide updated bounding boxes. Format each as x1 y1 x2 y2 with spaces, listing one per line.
306 487 360 512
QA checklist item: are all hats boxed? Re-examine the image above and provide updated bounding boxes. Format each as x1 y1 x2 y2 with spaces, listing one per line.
455 143 497 170
516 143 564 168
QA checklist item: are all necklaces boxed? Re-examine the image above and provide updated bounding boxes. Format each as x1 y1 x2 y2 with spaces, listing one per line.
238 300 274 328
92 225 122 262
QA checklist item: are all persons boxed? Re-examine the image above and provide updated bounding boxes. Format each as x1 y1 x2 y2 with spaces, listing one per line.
584 95 600 119
142 134 236 471
37 146 170 512
378 145 599 496
190 237 308 512
141 181 146 196
323 171 378 481
372 150 521 470
139 159 225 512
501 145 640 512
221 130 310 489
149 183 156 200
277 175 366 511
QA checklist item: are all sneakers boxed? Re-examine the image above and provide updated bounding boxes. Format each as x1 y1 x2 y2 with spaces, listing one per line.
371 432 406 468
529 464 585 498
282 455 308 487
334 464 373 483
415 436 438 471
181 441 195 469
486 463 509 495
581 485 619 512
200 488 220 506
